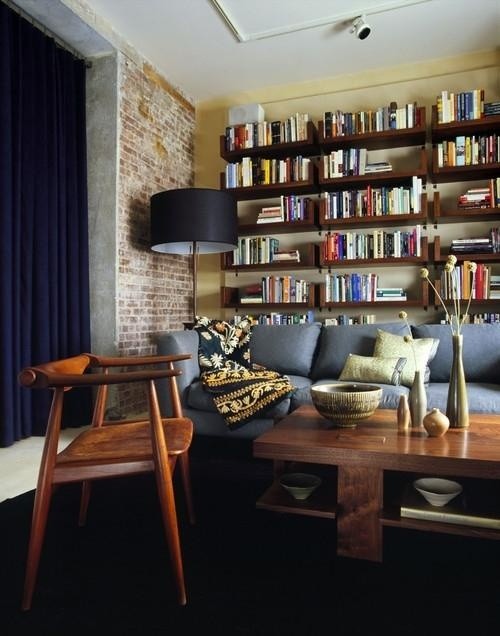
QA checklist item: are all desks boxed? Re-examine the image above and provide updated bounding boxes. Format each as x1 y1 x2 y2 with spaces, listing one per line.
252 402 500 566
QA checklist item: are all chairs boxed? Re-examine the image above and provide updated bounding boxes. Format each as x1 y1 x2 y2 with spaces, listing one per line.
18 353 194 610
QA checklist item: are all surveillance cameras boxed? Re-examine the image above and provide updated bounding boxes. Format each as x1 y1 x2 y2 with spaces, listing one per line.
351 17 372 41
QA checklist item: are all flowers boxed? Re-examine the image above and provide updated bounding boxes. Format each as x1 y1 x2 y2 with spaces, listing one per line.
418 255 478 335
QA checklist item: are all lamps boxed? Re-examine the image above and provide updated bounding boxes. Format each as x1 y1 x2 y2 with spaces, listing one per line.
150 187 239 322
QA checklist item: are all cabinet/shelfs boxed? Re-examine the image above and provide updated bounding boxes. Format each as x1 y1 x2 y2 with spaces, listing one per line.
219 106 500 310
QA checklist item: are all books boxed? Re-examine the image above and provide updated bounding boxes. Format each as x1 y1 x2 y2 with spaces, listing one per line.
233 237 300 265
323 225 420 260
256 195 304 224
450 227 500 255
325 176 422 220
225 157 313 188
226 113 316 152
324 313 376 325
441 312 499 324
326 273 408 303
241 276 309 303
436 90 500 123
441 264 500 299
324 148 392 178
438 136 500 167
458 178 500 209
234 310 314 324
323 102 425 138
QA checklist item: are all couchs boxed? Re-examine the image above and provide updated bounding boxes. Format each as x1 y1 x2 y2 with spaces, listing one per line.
152 314 500 454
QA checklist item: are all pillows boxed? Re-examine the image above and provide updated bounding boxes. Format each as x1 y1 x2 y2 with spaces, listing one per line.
411 321 499 383
339 354 408 390
250 321 323 377
311 321 413 379
372 328 440 387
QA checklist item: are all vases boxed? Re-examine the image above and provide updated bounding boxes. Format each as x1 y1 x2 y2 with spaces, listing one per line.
398 395 411 430
411 372 428 425
445 334 470 426
423 408 450 437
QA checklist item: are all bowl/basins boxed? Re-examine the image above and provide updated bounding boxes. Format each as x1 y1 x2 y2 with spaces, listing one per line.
279 472 322 500
310 382 383 429
413 478 464 507
